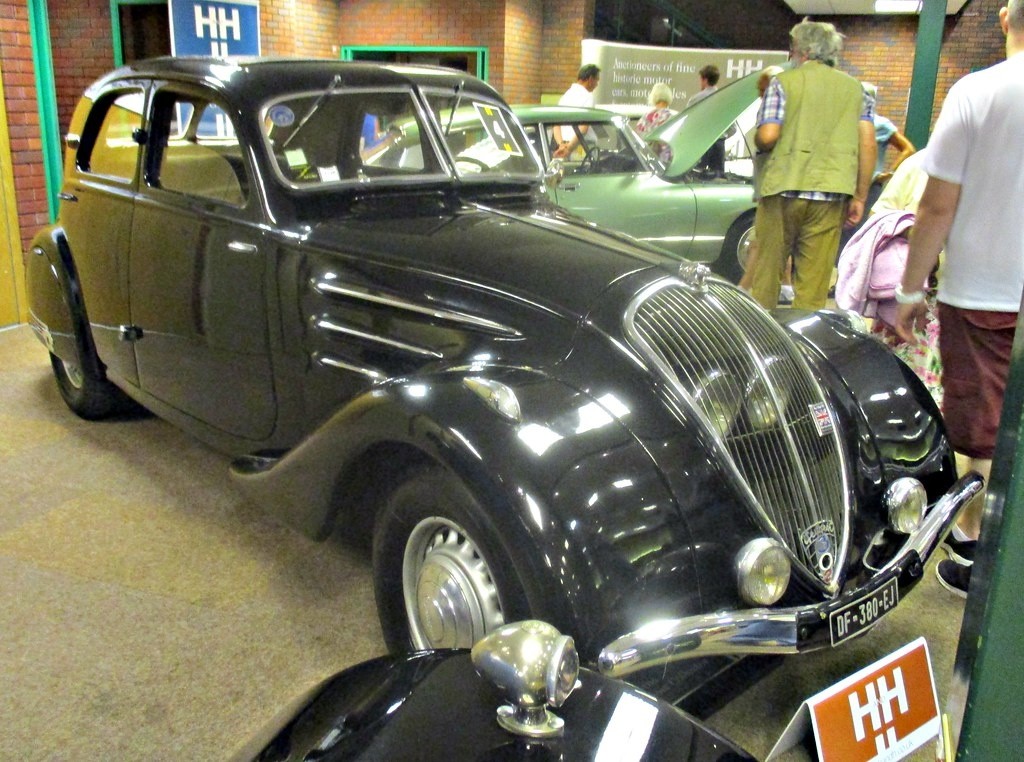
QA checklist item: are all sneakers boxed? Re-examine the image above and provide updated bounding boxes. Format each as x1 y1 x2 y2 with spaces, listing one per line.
936 559 972 600
940 528 977 566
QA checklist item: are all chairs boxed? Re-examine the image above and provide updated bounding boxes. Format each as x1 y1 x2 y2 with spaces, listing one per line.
162 153 252 205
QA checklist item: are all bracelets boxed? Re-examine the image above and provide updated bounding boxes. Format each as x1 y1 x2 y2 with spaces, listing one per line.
854 194 865 203
895 287 926 303
887 167 895 175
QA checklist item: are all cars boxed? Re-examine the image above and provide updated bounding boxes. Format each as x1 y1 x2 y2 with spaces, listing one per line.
280 55 784 281
23 56 988 709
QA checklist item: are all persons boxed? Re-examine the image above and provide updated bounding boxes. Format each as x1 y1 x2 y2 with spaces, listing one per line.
685 65 736 172
738 17 918 311
894 1 1024 599
553 64 600 162
637 82 679 161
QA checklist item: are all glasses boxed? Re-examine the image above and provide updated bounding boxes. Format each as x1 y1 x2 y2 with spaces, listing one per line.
790 43 799 51
